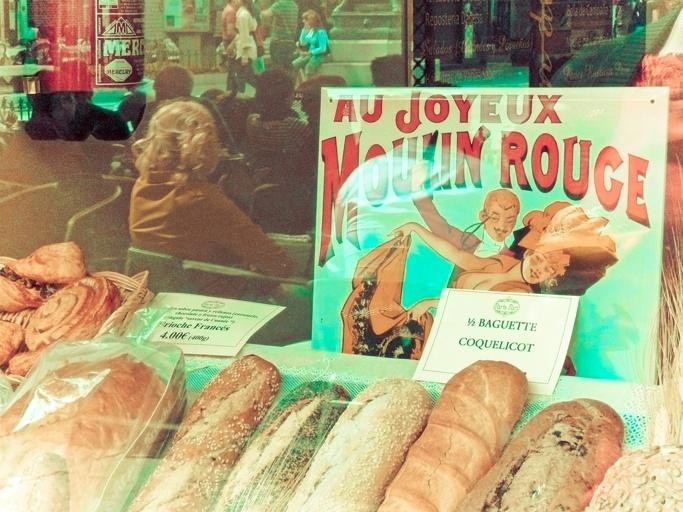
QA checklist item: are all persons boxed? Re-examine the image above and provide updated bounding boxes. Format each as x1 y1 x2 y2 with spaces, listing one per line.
339 197 616 376
0 0 405 345
411 189 520 287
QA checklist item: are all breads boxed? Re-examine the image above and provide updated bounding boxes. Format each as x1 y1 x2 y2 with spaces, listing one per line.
1 242 123 391
584 444 682 512
2 339 187 512
456 398 624 512
213 381 352 512
126 353 281 512
284 379 432 512
377 360 528 512
